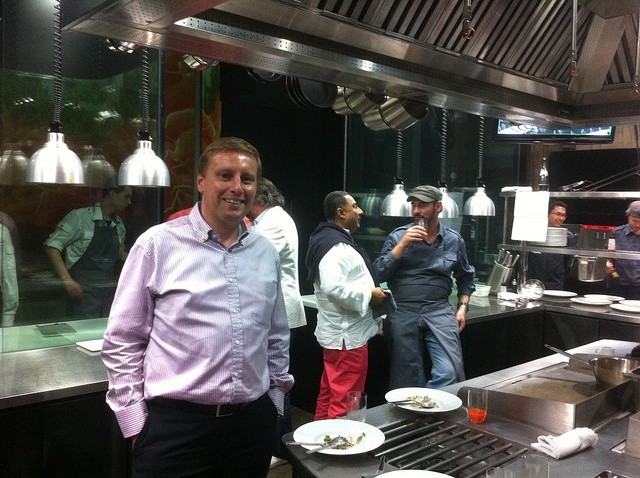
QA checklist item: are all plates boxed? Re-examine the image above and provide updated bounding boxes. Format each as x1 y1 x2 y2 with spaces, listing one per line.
584 294 626 301
611 303 638 313
570 298 611 305
544 227 568 247
294 418 385 455
384 387 463 415
544 289 577 297
619 300 639 306
375 469 452 478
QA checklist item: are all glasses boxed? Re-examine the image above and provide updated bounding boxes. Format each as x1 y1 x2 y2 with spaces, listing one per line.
552 212 566 218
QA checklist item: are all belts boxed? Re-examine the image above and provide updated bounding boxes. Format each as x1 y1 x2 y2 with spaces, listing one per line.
188 403 244 419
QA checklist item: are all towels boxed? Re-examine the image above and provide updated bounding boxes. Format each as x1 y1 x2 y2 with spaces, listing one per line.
530 427 598 459
502 186 533 192
510 189 550 243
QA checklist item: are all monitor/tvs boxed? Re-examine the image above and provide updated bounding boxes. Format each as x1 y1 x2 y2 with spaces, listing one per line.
492 118 615 144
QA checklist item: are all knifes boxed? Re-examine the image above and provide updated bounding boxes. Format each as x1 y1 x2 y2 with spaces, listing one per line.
498 248 521 268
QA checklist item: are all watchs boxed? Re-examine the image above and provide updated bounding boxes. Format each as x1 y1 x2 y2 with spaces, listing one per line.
457 302 469 312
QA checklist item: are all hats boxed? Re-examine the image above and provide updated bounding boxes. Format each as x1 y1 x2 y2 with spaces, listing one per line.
629 200 640 218
407 185 443 203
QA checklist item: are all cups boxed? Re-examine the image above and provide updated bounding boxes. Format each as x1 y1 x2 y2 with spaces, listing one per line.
416 219 429 231
595 346 615 355
467 389 487 424
346 391 368 423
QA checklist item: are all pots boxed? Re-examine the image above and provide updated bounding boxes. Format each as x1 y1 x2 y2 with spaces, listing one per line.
589 357 639 389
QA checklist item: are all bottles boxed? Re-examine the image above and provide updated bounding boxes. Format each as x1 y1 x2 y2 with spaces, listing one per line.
537 157 549 191
607 235 615 252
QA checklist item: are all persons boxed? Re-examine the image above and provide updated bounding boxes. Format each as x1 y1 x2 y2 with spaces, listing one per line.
42 176 130 319
305 191 389 419
102 136 295 477
1 224 19 326
606 201 640 298
372 185 477 398
241 176 308 461
529 201 577 290
0 211 19 280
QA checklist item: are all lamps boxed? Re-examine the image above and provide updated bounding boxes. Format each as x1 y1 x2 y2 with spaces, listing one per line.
463 116 496 218
26 0 84 184
437 108 459 218
381 131 411 217
118 47 170 188
1 77 30 185
82 36 117 190
362 195 385 218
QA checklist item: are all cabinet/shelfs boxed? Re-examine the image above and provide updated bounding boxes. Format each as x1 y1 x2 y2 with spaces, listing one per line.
466 312 545 379
1 393 131 478
545 311 639 356
498 191 640 291
